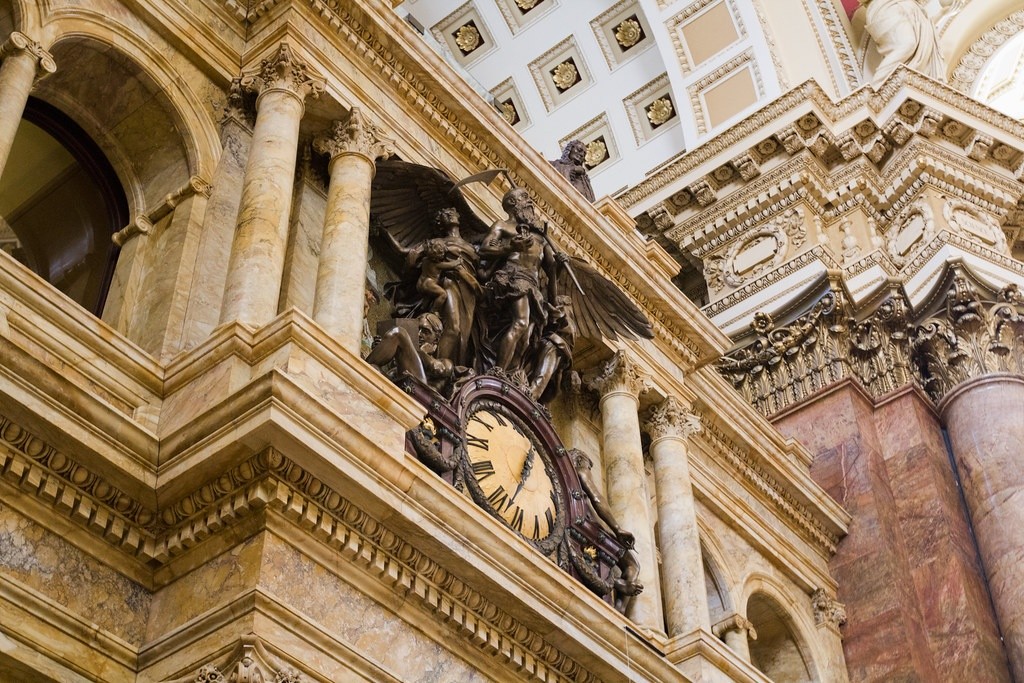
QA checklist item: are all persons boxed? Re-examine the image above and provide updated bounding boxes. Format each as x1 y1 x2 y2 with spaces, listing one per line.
550 138 596 204
370 186 645 614
860 0 945 93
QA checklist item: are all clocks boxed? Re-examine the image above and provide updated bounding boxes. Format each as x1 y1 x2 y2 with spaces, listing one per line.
464 394 572 556
